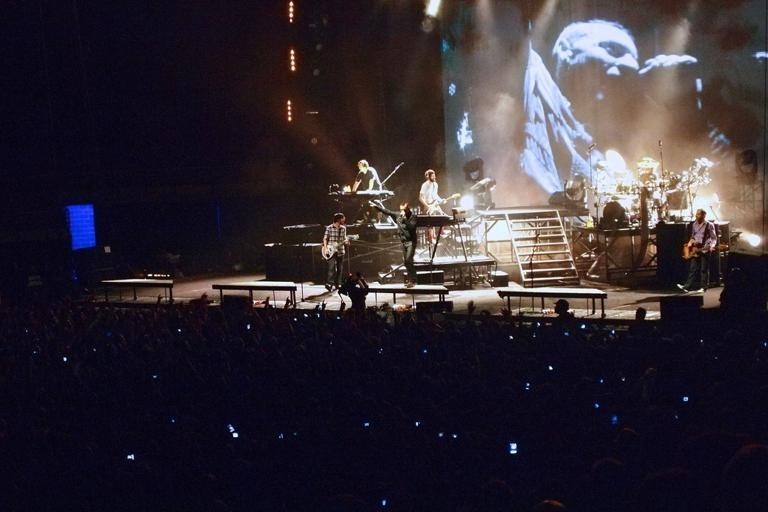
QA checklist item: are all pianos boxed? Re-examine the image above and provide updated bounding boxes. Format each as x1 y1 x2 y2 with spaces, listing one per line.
328 190 395 201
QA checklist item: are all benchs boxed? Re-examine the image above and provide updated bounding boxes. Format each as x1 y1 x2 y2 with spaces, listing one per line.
99 268 611 320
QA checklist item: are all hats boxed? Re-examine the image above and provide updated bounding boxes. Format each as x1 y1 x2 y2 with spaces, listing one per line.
552 299 569 306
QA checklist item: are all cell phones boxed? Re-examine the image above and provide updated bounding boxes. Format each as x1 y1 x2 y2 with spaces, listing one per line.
508 442 518 455
227 424 235 433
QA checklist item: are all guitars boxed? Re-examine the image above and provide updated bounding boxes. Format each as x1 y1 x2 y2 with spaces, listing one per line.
322 234 360 260
424 193 461 215
684 243 712 259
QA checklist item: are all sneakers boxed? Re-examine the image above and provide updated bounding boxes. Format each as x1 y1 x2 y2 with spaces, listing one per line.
677 283 689 292
404 280 415 287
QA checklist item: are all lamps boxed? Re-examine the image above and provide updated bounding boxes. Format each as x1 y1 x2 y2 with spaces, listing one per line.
424 0 442 17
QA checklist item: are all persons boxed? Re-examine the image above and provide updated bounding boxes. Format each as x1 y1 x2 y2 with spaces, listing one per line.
322 212 350 290
350 160 383 223
418 169 448 245
444 0 767 222
674 209 717 293
368 198 419 287
1 272 767 512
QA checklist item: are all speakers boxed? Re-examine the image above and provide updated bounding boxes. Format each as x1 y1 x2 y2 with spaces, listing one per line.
264 243 327 282
417 301 453 314
657 221 768 319
488 270 509 287
224 295 249 311
417 270 444 285
284 223 322 243
